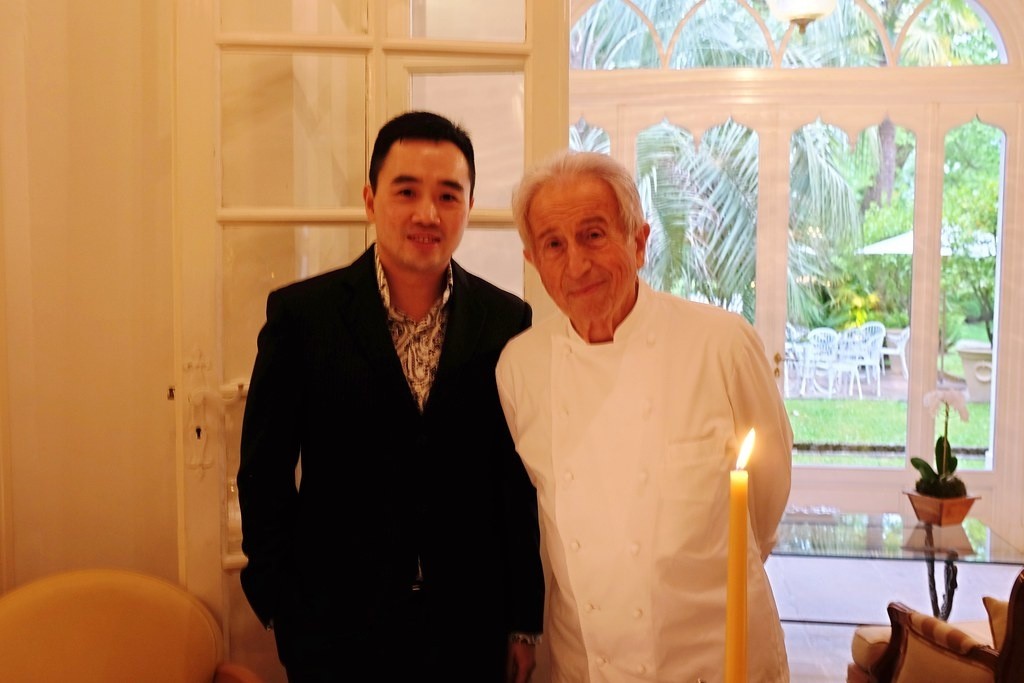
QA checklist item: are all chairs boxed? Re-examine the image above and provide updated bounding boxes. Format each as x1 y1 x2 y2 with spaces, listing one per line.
783 320 911 400
1 570 259 683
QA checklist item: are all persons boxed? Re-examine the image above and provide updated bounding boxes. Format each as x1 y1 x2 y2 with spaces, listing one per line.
237 111 544 683
495 152 793 683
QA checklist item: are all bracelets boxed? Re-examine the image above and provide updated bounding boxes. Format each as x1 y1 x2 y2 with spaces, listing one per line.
512 633 542 648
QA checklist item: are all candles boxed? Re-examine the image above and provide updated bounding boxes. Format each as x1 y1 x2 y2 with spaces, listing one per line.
726 466 750 683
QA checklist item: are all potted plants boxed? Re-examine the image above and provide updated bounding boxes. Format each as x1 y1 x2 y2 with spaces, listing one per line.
946 165 996 404
902 437 981 525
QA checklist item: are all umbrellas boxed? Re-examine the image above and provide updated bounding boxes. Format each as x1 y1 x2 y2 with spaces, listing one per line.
853 219 998 257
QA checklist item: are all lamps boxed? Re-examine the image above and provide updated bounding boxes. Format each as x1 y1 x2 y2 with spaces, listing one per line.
766 0 837 32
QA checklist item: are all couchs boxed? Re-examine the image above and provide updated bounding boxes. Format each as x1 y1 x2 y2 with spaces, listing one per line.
846 566 1024 683
